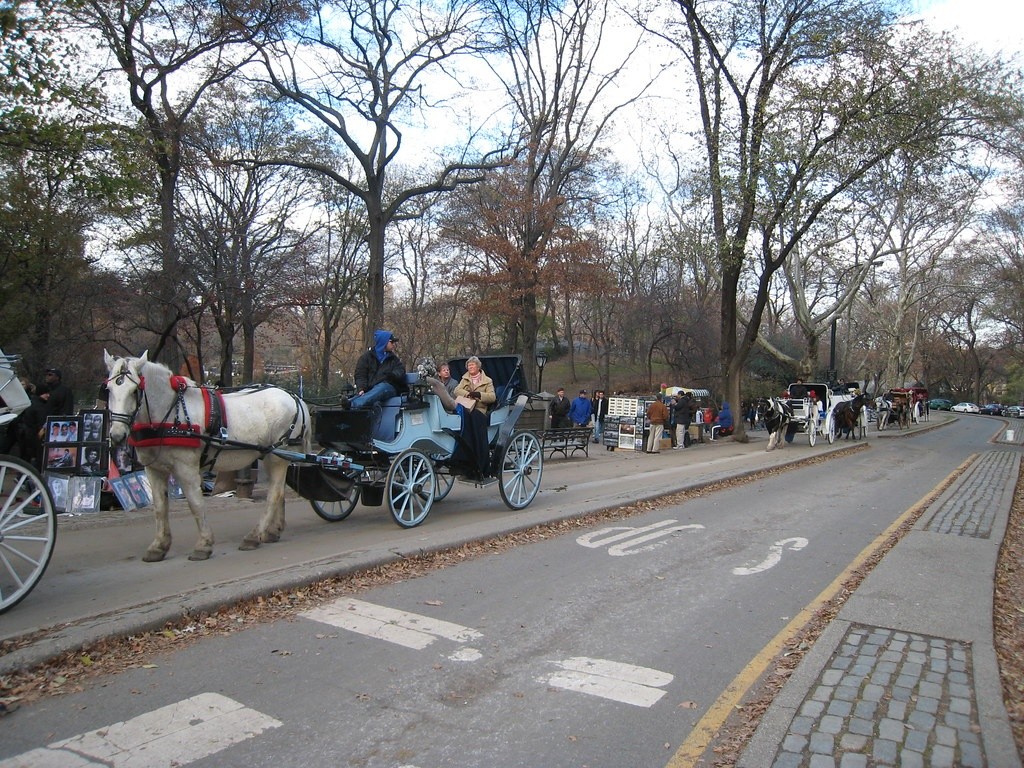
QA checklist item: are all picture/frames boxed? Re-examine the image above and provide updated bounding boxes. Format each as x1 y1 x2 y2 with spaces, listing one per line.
39 409 184 514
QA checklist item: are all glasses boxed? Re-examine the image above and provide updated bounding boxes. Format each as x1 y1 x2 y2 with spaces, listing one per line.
47 373 53 376
389 340 395 343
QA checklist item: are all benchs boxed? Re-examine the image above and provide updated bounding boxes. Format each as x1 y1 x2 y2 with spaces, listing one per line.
371 370 421 407
487 385 512 425
527 427 594 462
421 392 462 433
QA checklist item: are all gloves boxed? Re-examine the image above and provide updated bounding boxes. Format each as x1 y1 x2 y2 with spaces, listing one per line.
468 391 481 399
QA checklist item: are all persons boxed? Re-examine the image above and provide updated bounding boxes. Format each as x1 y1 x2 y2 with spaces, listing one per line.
49 414 101 441
70 479 95 509
435 364 458 400
711 401 733 440
812 394 825 417
757 404 762 423
338 329 409 411
669 390 696 449
548 388 571 442
115 448 131 471
696 406 705 422
646 393 669 452
449 355 496 478
48 445 100 473
567 389 594 442
886 389 894 401
48 479 66 506
826 372 847 395
590 390 609 444
0 367 73 509
703 399 719 433
748 402 756 430
789 379 809 400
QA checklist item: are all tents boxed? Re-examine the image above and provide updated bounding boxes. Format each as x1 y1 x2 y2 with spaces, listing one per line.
658 386 711 398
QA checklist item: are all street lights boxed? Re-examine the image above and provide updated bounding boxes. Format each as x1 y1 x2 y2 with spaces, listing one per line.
535 349 548 393
825 258 885 387
897 326 930 376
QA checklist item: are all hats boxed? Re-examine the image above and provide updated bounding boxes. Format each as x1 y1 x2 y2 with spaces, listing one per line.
388 334 399 342
580 389 587 394
47 368 62 378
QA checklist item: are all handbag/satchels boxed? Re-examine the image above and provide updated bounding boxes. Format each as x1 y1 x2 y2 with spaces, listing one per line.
455 394 476 413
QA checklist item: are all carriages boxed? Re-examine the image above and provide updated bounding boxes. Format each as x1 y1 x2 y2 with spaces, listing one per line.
103 348 547 564
756 380 929 452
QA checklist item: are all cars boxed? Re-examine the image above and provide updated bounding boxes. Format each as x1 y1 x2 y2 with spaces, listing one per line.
926 399 957 411
949 402 980 414
978 403 1003 416
1001 405 1024 419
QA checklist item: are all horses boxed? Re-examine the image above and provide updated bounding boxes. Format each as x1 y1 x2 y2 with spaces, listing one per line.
757 398 794 452
873 396 892 431
834 391 876 442
102 347 310 562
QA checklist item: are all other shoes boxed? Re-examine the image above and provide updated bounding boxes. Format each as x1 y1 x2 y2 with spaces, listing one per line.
710 438 717 441
592 439 599 444
673 446 683 450
647 451 660 454
341 396 352 411
